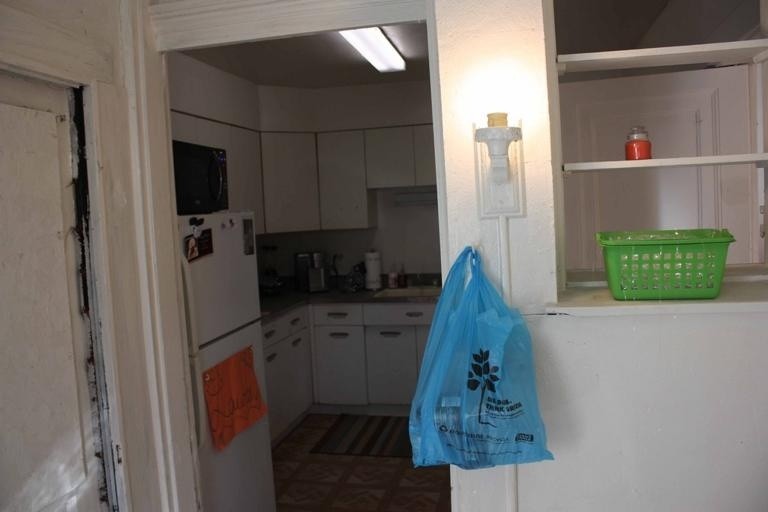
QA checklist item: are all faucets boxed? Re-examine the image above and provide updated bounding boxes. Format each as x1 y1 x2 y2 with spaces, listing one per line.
433 276 439 286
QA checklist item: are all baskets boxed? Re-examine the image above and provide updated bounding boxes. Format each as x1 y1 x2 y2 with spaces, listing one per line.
597 228 736 300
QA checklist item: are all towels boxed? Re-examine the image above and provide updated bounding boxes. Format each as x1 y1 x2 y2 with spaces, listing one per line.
205 345 269 451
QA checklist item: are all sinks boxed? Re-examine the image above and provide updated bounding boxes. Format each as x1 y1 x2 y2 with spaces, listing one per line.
373 287 442 298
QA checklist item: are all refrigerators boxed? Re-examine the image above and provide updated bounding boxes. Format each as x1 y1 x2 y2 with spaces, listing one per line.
176 209 278 512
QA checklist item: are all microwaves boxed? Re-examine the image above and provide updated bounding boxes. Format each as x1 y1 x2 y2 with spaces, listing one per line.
172 140 229 216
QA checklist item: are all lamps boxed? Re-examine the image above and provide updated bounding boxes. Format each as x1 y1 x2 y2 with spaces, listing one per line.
339 27 406 73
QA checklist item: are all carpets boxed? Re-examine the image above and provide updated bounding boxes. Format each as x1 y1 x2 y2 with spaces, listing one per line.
312 413 412 458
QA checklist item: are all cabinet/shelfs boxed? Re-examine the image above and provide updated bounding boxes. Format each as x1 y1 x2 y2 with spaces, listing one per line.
260 130 367 234
364 303 436 416
558 38 768 284
261 305 315 450
366 124 436 190
309 303 368 415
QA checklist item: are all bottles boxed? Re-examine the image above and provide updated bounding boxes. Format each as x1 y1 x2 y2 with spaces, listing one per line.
624 127 652 161
